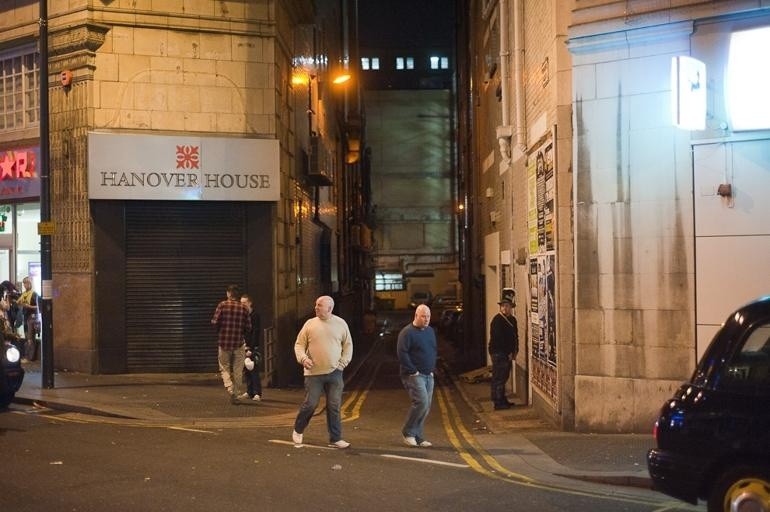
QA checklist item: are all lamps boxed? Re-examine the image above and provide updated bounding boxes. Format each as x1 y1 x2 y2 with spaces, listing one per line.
331 59 351 84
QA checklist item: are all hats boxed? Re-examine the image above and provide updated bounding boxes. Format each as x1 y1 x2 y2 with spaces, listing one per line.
498 290 516 307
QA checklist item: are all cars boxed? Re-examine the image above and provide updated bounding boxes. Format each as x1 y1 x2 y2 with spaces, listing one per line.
410 291 463 333
646 296 769 511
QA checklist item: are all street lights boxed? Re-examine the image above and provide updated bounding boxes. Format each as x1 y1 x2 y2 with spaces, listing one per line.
292 73 352 221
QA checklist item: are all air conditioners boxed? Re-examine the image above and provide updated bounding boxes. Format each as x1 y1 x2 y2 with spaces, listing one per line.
309 135 334 186
352 224 373 252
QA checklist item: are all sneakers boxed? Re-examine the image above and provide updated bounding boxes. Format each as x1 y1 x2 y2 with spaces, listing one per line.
292 429 303 444
243 392 250 398
252 395 263 401
495 402 514 409
230 392 240 404
236 394 242 401
419 441 432 447
328 440 351 449
401 432 417 446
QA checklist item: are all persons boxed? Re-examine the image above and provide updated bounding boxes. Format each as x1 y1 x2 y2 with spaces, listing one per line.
16 276 39 361
210 283 249 405
488 303 519 409
292 295 353 449
397 303 437 447
240 293 262 401
